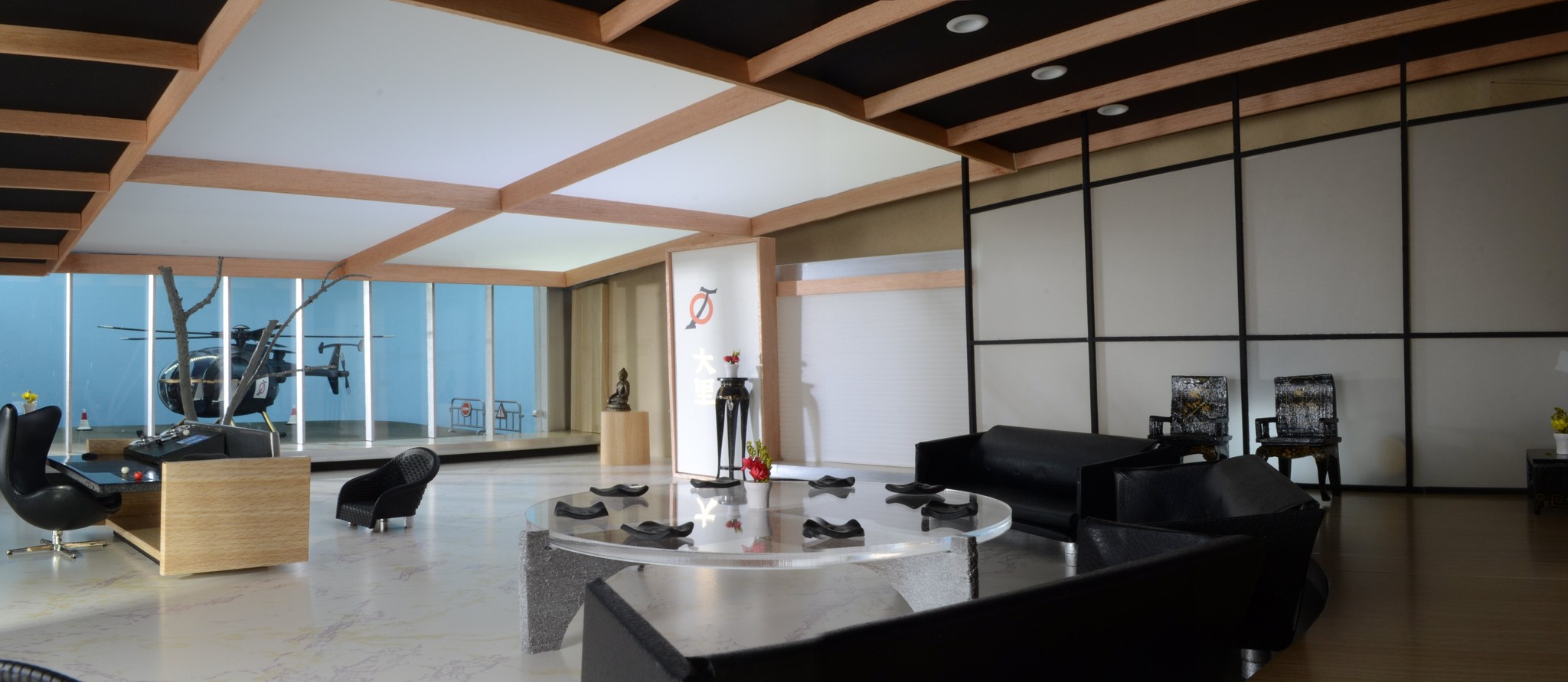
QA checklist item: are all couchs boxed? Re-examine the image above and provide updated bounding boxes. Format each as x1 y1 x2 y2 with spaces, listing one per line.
577 521 1250 682
1113 452 1326 681
915 424 1161 545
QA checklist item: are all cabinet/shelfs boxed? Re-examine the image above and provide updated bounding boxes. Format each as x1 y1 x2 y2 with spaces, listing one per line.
84 438 312 577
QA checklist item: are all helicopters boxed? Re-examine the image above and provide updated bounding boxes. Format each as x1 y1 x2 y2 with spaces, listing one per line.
97 321 393 436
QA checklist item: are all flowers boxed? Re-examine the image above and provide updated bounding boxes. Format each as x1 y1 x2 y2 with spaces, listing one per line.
1550 406 1568 434
723 349 741 365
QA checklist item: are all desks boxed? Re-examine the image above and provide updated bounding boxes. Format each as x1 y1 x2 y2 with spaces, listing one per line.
43 453 164 494
1525 448 1568 515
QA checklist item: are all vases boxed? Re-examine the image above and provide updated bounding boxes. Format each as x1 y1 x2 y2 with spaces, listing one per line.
1553 433 1568 455
723 364 740 378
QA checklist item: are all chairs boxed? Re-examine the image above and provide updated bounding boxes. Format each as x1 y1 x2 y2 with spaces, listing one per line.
0 403 123 560
1256 374 1344 501
335 447 440 533
1146 375 1233 463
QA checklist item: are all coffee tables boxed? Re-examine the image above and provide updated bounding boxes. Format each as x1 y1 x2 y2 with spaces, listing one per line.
515 475 1015 656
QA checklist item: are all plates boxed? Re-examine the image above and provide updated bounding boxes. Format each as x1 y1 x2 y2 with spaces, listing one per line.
802 516 864 539
621 521 695 540
555 501 609 519
590 483 650 497
691 476 741 488
885 482 946 495
922 499 978 520
808 475 855 489
